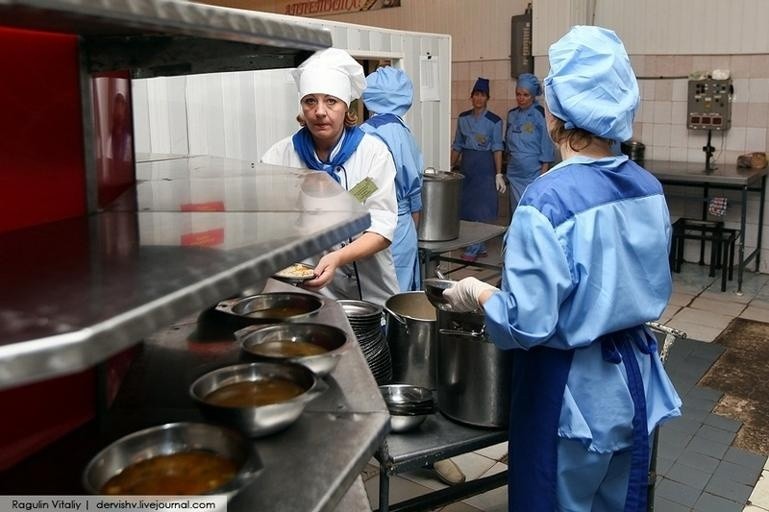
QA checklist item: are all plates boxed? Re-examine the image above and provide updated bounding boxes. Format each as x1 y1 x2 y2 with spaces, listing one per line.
338 300 392 382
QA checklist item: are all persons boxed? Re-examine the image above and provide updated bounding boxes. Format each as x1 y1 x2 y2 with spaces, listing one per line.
436 24 684 511
505 74 555 217
360 64 424 294
259 46 463 490
452 77 506 264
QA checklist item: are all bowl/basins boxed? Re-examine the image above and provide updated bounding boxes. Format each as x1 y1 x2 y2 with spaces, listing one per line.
274 263 316 283
82 421 262 500
190 363 327 437
379 385 432 432
216 291 326 321
421 279 459 311
235 324 351 377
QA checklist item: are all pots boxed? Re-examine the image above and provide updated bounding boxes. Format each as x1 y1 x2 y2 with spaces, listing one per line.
386 292 436 390
419 170 465 241
625 141 645 162
435 309 511 428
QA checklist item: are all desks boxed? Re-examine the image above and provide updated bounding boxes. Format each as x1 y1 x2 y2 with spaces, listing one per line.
385 416 510 512
415 222 507 293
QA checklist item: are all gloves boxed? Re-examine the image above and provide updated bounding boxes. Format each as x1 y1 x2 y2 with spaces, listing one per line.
441 276 498 313
496 174 507 193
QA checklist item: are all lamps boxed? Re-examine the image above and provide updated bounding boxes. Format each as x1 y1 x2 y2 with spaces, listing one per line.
640 156 769 296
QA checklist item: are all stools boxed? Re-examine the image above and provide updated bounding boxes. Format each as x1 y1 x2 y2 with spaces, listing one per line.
670 217 742 292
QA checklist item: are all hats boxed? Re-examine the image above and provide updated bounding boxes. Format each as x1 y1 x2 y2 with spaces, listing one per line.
362 66 413 116
473 77 489 94
517 73 542 96
291 47 367 110
544 25 640 143
